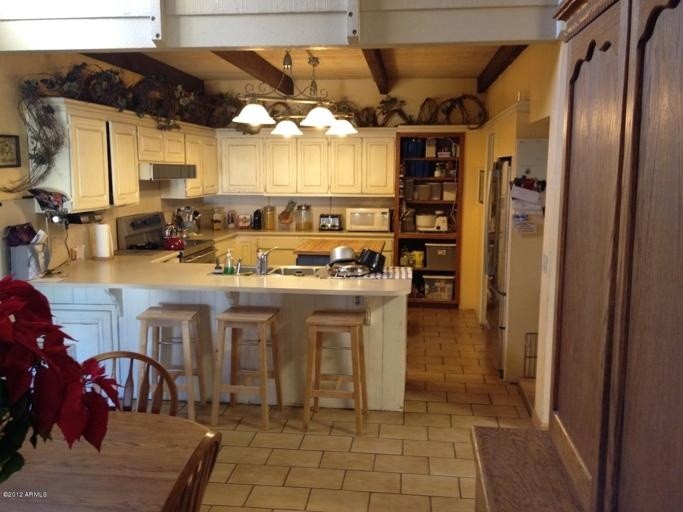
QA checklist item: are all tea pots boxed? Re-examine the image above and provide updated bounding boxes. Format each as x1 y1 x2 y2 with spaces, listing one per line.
164 228 185 249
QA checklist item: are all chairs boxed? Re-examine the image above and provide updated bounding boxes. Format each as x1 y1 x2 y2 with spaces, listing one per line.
136 306 203 424
155 431 221 512
210 306 282 430
75 352 177 417
303 309 367 437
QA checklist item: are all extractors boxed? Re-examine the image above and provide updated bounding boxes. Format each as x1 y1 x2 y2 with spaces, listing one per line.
139 163 196 182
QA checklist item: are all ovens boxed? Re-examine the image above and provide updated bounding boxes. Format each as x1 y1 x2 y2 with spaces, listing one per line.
180 247 218 266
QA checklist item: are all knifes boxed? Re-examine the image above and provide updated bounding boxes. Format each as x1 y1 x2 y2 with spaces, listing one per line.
174 205 199 235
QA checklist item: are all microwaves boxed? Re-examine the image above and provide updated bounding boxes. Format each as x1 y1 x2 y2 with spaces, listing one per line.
344 208 391 232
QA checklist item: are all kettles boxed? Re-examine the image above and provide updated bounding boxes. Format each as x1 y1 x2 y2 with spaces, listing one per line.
90 223 114 262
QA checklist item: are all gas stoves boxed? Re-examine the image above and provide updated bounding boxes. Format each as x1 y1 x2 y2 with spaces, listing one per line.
127 235 213 251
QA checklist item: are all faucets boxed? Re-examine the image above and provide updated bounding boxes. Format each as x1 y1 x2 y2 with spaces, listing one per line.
215 250 231 269
257 246 277 274
236 257 243 273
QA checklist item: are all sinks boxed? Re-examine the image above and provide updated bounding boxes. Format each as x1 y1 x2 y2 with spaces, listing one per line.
234 267 273 273
271 268 321 276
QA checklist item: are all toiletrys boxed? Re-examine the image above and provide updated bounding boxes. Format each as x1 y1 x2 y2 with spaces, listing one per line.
224 249 234 275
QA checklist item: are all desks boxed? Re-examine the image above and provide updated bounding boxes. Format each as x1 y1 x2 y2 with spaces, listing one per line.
0 407 211 512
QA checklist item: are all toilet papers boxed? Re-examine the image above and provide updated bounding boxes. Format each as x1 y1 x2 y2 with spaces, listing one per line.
89 224 116 258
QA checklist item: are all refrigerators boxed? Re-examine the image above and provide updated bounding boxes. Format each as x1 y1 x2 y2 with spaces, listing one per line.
482 138 546 385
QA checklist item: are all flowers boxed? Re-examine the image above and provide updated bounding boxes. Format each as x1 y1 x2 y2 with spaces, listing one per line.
0 273 121 484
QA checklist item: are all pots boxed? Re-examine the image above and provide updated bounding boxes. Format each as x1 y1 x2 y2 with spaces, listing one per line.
329 246 356 266
326 262 371 279
356 247 385 274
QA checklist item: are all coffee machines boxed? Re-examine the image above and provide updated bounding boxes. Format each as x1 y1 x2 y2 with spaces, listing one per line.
318 213 341 231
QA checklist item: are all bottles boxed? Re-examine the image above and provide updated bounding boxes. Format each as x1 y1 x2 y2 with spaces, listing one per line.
199 202 314 232
398 135 460 269
255 248 266 275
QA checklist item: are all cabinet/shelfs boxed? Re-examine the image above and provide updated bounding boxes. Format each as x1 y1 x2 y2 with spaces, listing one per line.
219 127 266 197
233 234 256 266
136 113 184 164
215 236 233 263
397 132 463 309
158 124 219 199
264 126 325 197
325 126 395 199
22 99 140 215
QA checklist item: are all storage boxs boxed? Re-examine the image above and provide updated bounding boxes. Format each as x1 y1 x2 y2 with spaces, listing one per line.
421 274 455 301
424 241 455 270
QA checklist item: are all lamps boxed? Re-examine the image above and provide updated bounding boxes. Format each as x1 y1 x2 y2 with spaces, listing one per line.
268 58 360 138
232 52 335 132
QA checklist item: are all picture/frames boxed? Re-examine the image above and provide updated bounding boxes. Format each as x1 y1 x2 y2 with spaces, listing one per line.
0 135 20 168
